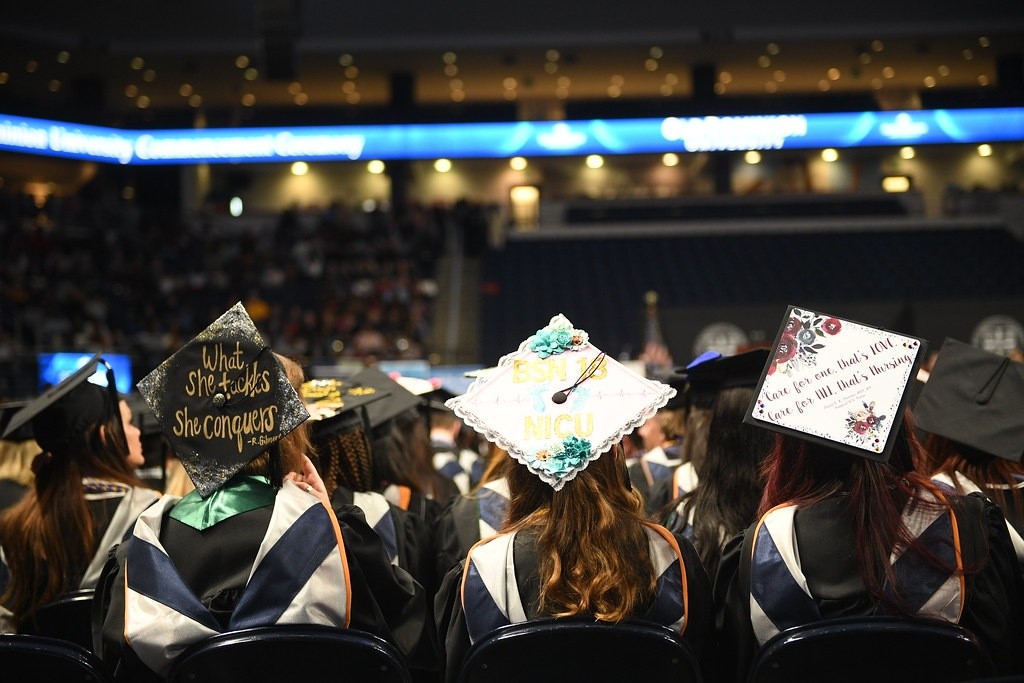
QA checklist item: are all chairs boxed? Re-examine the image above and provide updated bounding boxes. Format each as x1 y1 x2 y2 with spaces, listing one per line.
0 588 980 683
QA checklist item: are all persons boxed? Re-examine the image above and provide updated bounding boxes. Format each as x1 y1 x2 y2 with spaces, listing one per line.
90 304 432 683
0 352 163 654
721 304 1024 683
0 182 484 404
432 313 712 683
0 337 1024 683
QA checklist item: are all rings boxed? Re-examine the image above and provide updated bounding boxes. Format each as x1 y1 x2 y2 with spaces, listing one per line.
304 485 312 493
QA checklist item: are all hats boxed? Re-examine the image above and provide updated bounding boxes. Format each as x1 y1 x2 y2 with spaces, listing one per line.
138 302 309 499
909 334 1024 467
741 304 931 460
1 352 134 459
300 362 454 440
441 313 677 491
678 348 771 396
